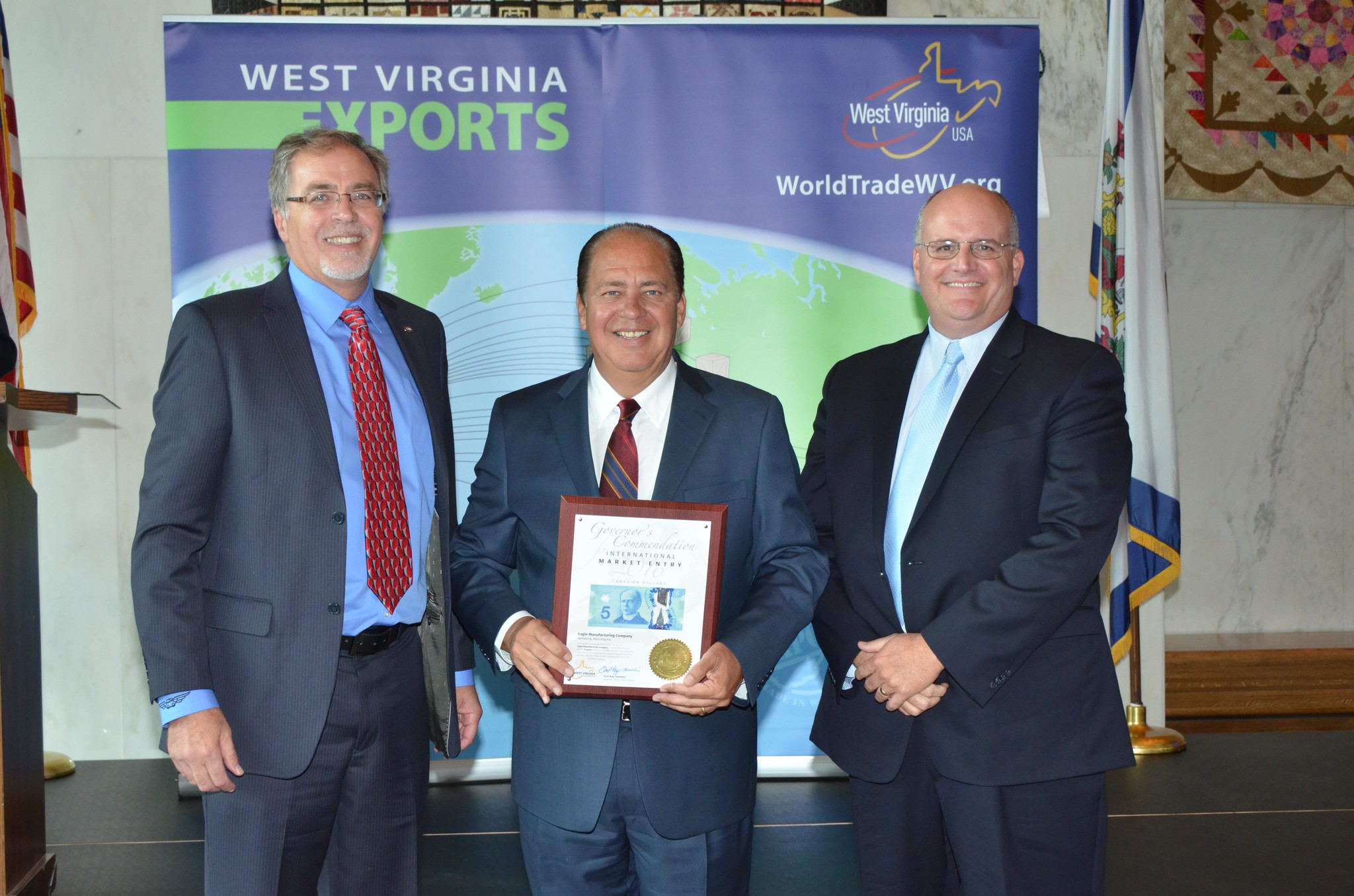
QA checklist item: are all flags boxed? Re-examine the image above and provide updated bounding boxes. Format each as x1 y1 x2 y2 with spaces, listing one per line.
1087 0 1180 666
1 2 40 485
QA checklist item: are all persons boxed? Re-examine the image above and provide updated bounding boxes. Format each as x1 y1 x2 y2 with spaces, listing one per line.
131 130 485 896
450 222 834 896
800 183 1136 896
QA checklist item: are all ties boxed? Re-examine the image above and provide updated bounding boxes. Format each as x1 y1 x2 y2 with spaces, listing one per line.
599 398 640 501
338 306 413 615
883 342 964 634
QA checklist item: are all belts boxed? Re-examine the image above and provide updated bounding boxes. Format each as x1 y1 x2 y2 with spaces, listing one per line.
341 621 410 657
620 702 631 721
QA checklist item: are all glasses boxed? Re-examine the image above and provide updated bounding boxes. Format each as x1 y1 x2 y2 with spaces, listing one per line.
915 239 1014 260
285 189 387 210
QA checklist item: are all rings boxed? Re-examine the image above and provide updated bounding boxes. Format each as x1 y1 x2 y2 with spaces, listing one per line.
880 685 892 696
701 708 708 715
